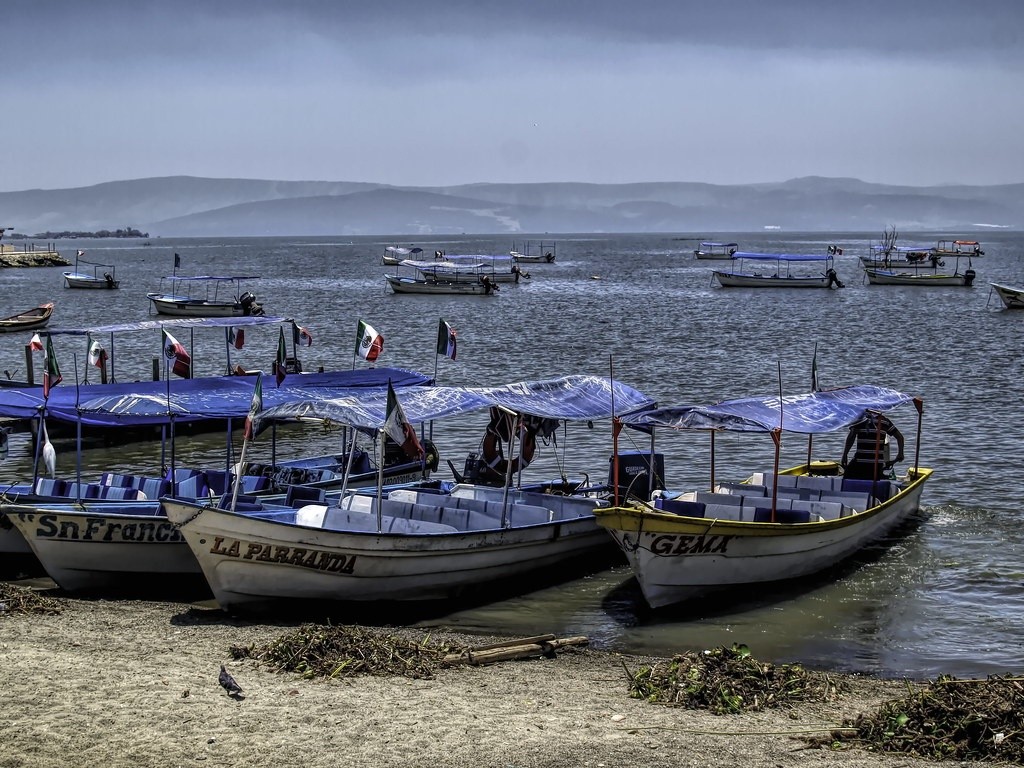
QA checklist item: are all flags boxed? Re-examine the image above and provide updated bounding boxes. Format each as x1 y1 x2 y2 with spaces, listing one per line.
44 333 62 401
86 338 108 369
354 320 384 365
382 379 426 460
29 333 44 352
276 326 287 388
162 330 190 380
436 317 457 361
174 253 181 267
291 321 312 348
227 326 245 350
76 249 84 257
244 376 265 443
827 245 843 256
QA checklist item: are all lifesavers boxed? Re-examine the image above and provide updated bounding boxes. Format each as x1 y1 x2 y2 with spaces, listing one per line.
483 423 536 473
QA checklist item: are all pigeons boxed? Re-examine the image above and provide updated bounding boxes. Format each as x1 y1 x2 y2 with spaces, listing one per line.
218 664 244 697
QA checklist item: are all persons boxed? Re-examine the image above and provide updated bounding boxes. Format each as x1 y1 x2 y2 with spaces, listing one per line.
842 409 905 480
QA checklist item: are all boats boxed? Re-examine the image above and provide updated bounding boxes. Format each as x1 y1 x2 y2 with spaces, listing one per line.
858 245 945 268
146 275 265 316
33 316 313 442
383 260 500 295
711 244 846 289
62 249 120 289
0 303 55 333
930 239 986 257
510 251 556 263
990 283 1024 309
421 254 532 282
591 343 933 608
864 257 976 286
694 242 738 260
159 375 659 617
0 318 456 595
382 246 425 265
